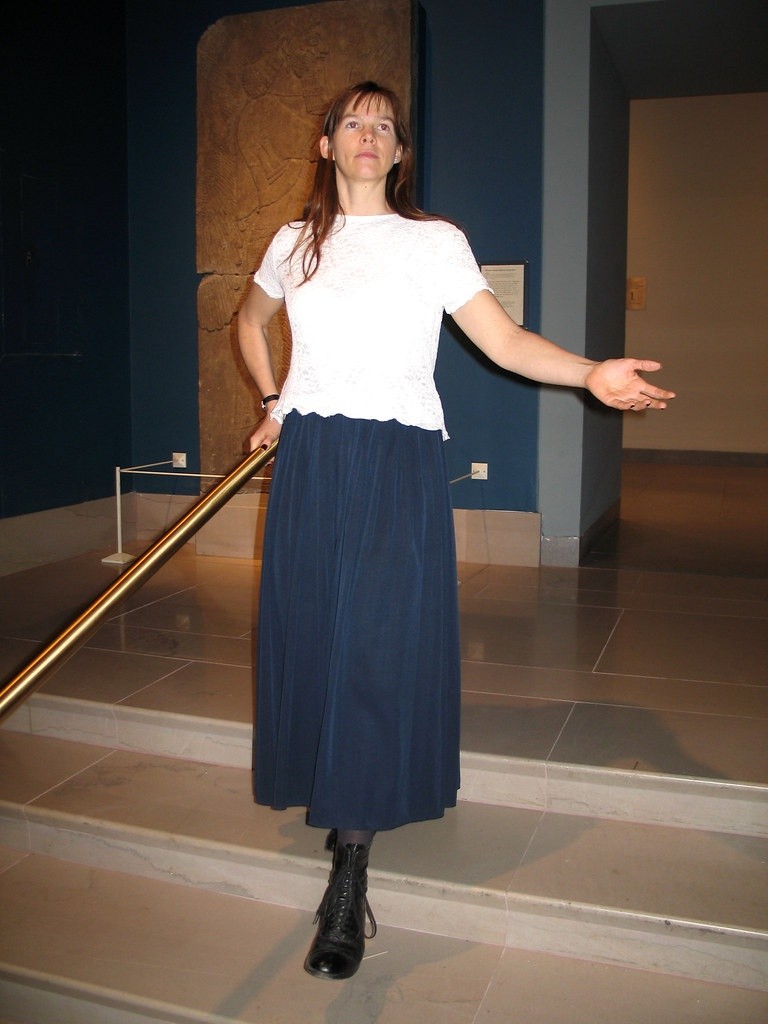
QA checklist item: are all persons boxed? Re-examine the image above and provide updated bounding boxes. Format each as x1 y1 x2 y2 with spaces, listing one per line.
238 82 676 980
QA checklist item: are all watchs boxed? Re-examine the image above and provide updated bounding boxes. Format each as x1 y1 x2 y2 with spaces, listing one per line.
260 394 279 410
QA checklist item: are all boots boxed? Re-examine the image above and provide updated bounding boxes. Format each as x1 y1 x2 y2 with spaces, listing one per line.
322 830 337 851
303 842 378 980
